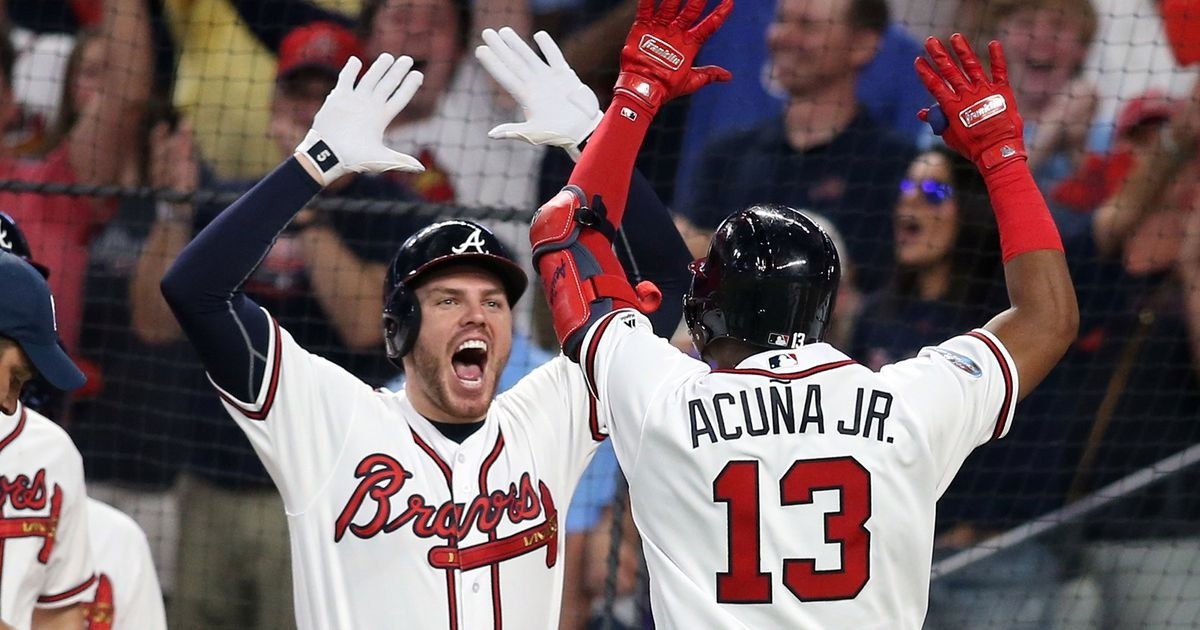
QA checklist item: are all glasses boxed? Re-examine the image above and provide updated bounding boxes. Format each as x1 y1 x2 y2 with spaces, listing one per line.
897 180 957 208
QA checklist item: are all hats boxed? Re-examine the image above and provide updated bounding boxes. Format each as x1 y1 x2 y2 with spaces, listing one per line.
0 249 87 391
1117 88 1178 135
277 21 370 79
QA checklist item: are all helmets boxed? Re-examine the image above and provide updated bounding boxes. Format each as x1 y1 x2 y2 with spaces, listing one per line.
682 204 842 362
384 218 527 331
0 212 50 280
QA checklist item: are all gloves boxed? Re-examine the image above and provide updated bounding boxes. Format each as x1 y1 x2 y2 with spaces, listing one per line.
476 27 606 164
612 0 735 116
296 50 427 187
914 33 1029 172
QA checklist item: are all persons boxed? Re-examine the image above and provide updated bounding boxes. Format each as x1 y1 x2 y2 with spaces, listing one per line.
2 0 1198 630
531 0 1079 629
160 26 695 630
0 209 167 630
